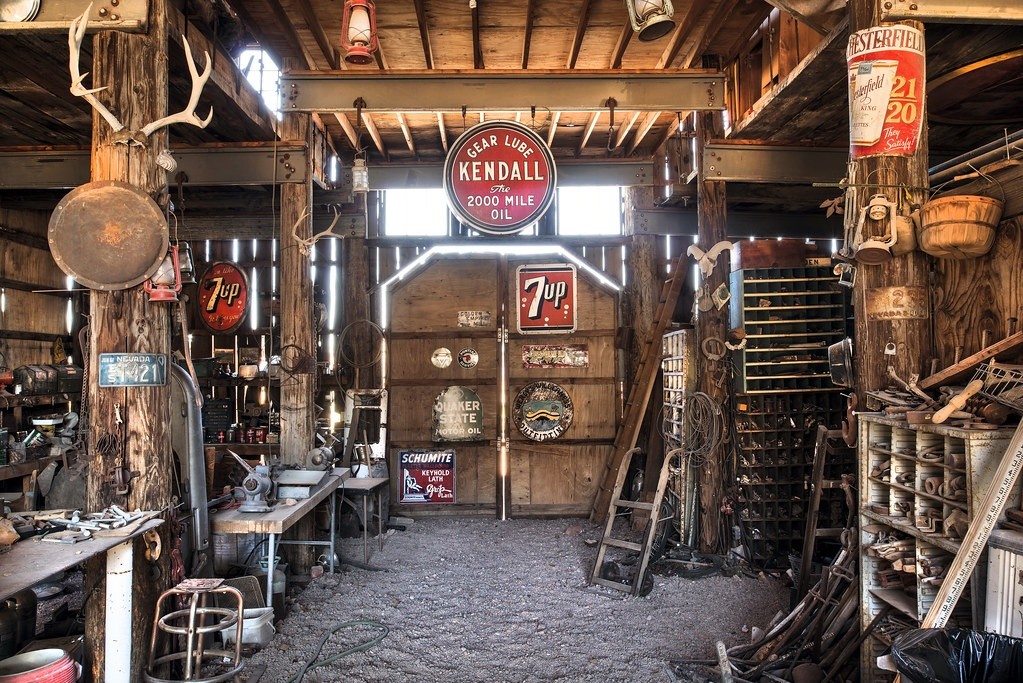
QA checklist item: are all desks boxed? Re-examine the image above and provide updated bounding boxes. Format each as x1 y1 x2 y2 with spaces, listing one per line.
335 476 389 566
208 467 352 619
1 508 165 682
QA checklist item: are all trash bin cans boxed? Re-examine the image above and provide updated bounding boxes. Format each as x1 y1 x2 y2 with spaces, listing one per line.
892 627 1023 683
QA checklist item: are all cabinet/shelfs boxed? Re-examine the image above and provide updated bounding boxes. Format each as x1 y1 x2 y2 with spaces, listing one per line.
661 328 695 548
730 238 859 569
860 412 1023 683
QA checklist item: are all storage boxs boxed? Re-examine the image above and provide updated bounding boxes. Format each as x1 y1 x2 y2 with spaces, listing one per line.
8 442 51 464
50 364 84 393
13 364 58 396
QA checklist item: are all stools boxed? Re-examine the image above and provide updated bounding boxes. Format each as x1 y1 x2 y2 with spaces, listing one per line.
141 577 245 683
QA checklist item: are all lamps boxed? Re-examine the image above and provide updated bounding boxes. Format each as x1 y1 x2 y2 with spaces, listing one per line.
341 0 379 65
625 0 676 45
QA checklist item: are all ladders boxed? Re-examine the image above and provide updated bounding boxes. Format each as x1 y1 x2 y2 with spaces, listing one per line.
586 252 693 527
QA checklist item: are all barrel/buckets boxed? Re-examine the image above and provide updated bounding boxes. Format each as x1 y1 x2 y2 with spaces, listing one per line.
0 647 82 683
